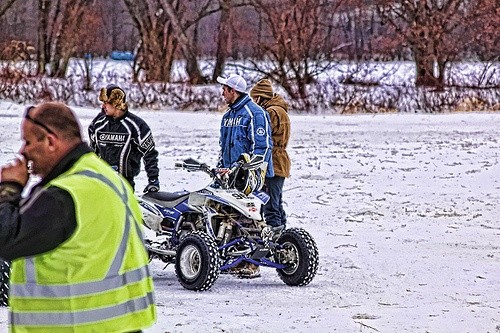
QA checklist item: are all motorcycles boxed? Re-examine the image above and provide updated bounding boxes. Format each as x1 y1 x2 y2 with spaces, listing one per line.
138 156 319 292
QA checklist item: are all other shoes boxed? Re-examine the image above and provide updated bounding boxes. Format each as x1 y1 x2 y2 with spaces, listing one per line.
227 266 242 274
239 263 260 278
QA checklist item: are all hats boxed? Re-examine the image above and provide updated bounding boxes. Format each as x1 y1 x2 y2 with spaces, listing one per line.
217 75 247 93
99 84 127 111
250 80 273 98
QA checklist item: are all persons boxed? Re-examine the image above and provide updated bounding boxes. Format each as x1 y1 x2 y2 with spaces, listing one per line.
87 84 161 193
0 101 157 333
250 78 290 232
215 74 275 279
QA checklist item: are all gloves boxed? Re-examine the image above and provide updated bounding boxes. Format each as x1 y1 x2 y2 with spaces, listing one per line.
143 181 159 193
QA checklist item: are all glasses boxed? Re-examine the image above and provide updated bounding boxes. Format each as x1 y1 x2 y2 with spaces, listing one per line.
23 106 59 139
